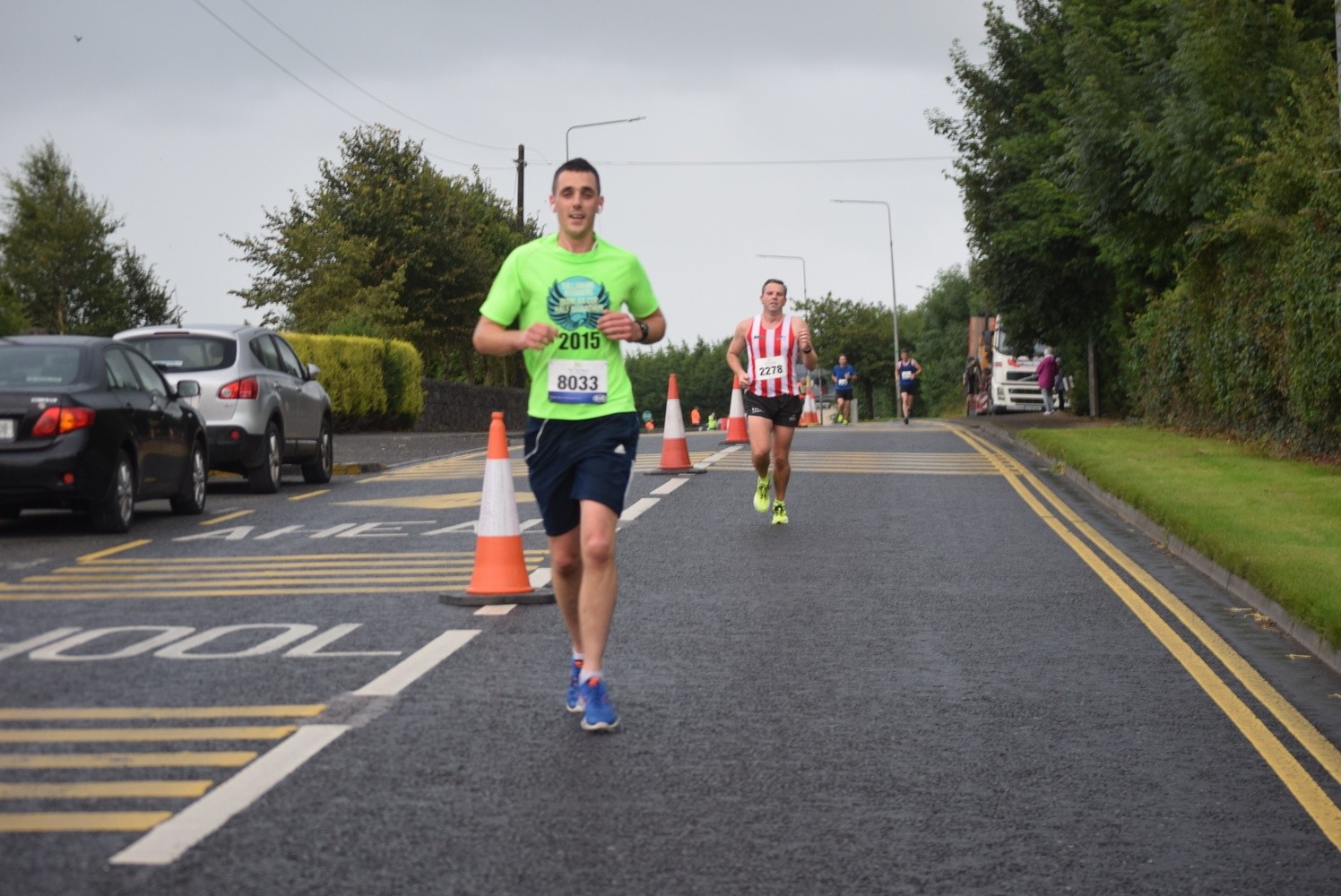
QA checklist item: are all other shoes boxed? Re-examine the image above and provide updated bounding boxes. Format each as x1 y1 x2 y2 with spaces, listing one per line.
843 419 848 425
838 413 844 423
904 418 909 425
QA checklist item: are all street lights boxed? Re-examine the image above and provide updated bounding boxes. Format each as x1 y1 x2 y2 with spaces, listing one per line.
831 198 902 419
755 254 807 324
565 116 646 162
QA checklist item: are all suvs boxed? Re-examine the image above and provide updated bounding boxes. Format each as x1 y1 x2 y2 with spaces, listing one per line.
112 321 334 494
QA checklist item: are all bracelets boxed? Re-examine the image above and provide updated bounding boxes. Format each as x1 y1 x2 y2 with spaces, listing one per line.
804 348 811 353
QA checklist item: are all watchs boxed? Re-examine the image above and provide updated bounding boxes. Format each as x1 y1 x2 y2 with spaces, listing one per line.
634 320 649 343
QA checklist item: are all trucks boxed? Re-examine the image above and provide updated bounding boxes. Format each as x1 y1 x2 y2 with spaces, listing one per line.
967 311 1075 416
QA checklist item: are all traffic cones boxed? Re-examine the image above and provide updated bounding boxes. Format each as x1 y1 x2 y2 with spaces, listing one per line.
797 389 819 427
642 373 708 475
438 412 556 605
718 374 750 445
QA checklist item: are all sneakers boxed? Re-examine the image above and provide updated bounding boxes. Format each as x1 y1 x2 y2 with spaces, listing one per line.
578 675 620 731
753 475 770 513
565 658 586 713
772 498 789 526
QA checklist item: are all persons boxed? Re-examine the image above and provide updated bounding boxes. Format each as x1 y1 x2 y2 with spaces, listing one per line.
831 353 857 424
473 158 665 731
691 405 701 427
726 279 819 524
708 410 718 430
645 418 654 430
796 377 806 398
1036 348 1066 416
895 347 921 424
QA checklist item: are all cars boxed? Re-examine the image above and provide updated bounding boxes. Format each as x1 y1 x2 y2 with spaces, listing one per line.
0 334 210 534
